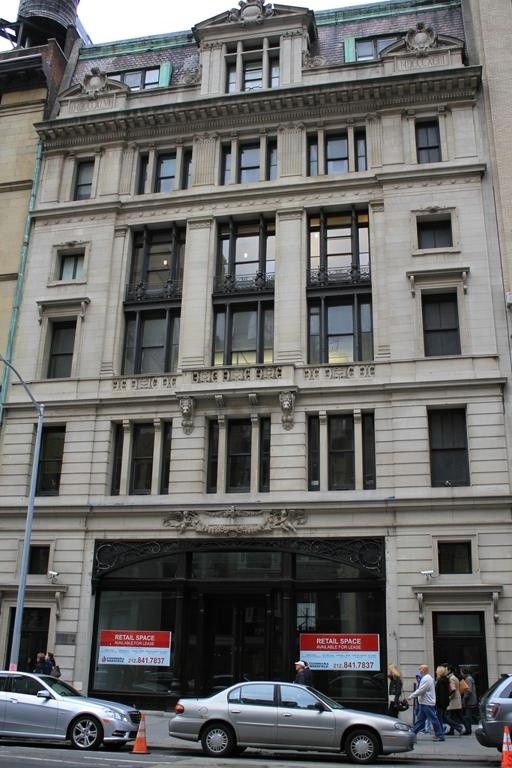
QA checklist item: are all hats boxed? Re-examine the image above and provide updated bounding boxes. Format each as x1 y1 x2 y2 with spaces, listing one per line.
293 660 304 666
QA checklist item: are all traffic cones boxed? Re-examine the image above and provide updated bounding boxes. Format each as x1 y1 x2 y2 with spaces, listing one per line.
129 711 151 753
501 726 512 767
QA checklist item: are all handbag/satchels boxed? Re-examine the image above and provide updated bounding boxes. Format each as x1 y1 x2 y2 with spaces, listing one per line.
447 678 456 694
395 699 410 709
50 665 61 678
457 679 469 697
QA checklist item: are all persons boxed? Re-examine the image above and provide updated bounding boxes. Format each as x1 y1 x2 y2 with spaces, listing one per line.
434 666 462 737
316 264 327 287
32 652 49 676
44 652 57 676
254 271 265 291
223 275 237 295
385 664 403 718
460 666 478 736
443 666 462 736
350 263 360 283
415 672 432 735
135 278 144 303
294 661 307 686
406 665 444 743
163 277 175 299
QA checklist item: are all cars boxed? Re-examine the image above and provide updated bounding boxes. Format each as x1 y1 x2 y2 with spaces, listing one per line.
170 674 254 697
93 670 168 693
322 674 386 699
169 679 418 763
0 669 141 748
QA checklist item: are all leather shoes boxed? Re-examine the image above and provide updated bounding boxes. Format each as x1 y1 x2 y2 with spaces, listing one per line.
410 728 472 741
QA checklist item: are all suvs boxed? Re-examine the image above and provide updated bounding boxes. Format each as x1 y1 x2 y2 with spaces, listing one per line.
475 672 512 750
